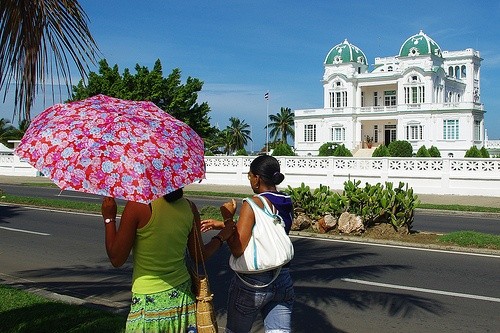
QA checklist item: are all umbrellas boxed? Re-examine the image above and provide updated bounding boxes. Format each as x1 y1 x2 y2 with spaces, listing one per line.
14 93 207 204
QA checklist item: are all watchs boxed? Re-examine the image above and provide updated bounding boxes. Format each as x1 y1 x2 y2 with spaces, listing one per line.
104 218 117 223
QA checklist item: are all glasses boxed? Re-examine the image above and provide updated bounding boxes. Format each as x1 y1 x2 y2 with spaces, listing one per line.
248 171 257 180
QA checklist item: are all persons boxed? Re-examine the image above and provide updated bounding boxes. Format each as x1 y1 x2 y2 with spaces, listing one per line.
101 186 235 333
448 153 454 158
368 143 372 149
200 155 295 333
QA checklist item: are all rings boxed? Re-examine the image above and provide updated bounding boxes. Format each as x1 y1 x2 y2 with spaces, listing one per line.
233 227 235 230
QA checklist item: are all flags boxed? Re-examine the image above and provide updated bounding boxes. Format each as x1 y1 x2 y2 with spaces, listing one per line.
264 93 269 101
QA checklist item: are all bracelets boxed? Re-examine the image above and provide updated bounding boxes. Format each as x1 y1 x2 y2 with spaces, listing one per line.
223 218 232 224
211 236 223 247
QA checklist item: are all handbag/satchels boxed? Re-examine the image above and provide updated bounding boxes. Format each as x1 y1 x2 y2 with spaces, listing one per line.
229 193 295 289
188 265 220 333
